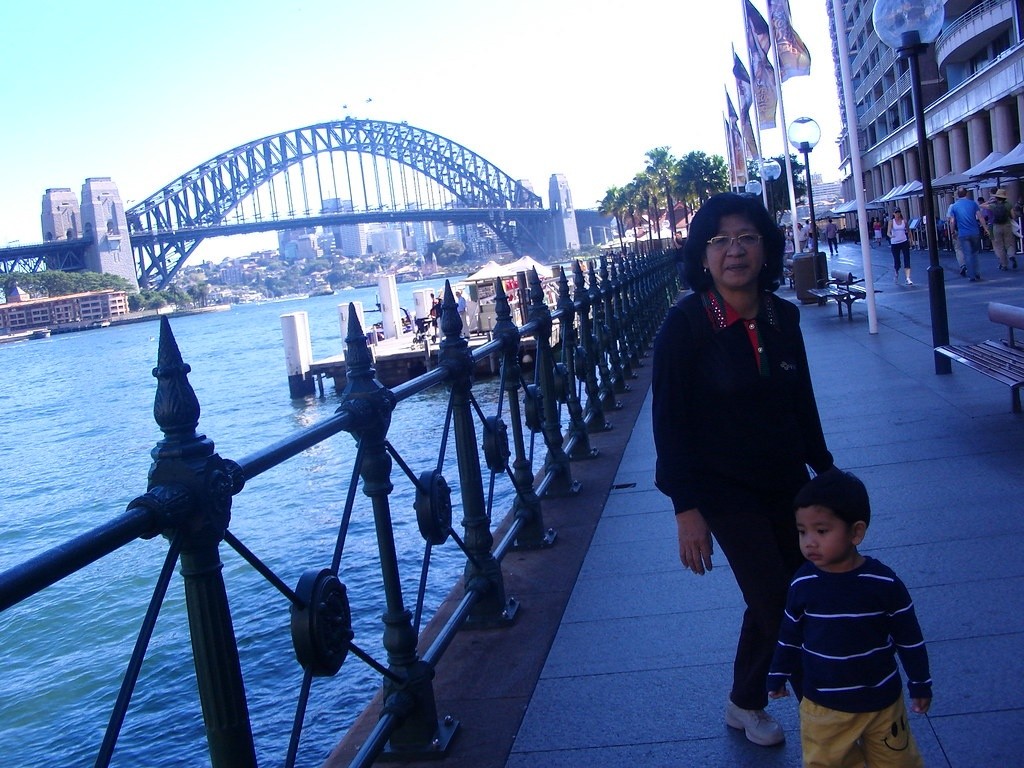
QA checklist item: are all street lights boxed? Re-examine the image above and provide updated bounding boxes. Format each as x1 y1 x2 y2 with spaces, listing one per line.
872 0 953 377
759 158 782 227
786 116 822 253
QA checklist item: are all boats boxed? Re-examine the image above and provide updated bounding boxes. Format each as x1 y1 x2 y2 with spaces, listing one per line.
308 281 334 298
26 328 53 341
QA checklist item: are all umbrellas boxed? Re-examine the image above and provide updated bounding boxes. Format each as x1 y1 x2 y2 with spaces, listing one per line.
600 228 675 249
931 171 980 203
815 199 883 222
868 180 929 250
961 142 1024 189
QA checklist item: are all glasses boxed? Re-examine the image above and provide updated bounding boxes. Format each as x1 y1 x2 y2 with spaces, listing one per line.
894 210 901 213
706 234 764 246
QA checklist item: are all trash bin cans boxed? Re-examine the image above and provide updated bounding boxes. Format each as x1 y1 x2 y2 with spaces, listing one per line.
793 251 829 305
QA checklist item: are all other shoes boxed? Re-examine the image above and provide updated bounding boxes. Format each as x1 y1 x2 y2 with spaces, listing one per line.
894 277 899 284
1010 257 1018 268
969 274 981 280
997 265 1008 271
904 278 913 285
960 266 967 275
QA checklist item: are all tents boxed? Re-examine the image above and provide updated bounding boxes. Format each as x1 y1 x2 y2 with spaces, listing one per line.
460 255 553 312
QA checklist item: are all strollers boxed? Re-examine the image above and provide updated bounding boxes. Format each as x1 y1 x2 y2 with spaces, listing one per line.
413 312 439 344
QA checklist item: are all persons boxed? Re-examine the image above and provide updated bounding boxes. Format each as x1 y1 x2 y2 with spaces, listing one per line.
855 213 889 245
780 224 794 240
797 223 807 252
826 218 838 256
936 217 944 249
887 207 913 286
977 186 1024 272
946 187 990 281
455 290 471 339
608 250 616 257
804 220 813 250
673 231 687 247
653 196 839 745
427 293 438 318
580 259 587 271
765 471 933 767
429 294 442 346
401 318 410 326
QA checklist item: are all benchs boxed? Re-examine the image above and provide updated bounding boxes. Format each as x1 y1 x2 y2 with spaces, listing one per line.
807 270 884 322
934 301 1024 413
782 251 794 290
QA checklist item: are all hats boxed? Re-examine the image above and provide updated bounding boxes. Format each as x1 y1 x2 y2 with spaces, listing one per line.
893 207 900 213
991 189 1009 198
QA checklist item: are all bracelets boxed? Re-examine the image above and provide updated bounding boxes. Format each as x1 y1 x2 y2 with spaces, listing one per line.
951 231 955 235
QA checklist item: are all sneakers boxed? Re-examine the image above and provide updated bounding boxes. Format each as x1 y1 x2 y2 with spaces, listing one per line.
724 691 785 745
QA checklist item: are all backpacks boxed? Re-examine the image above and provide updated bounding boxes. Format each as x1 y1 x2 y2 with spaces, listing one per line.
992 201 1008 225
